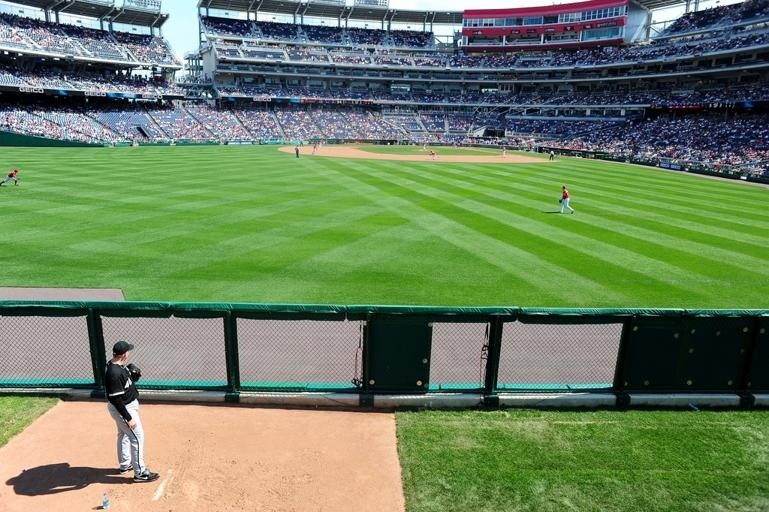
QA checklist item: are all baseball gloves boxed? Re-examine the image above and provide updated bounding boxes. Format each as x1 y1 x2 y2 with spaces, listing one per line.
559 199 562 203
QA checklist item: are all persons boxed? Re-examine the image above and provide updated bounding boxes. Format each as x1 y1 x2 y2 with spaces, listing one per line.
105 341 160 482
0 1 769 187
559 185 574 214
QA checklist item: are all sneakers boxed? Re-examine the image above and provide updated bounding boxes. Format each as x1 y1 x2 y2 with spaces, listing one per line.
134 471 159 482
119 465 134 474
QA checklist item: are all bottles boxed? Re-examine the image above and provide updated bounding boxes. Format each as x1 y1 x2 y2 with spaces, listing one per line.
102 492 111 510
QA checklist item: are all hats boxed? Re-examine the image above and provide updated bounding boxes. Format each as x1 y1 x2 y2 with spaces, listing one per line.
113 341 132 354
